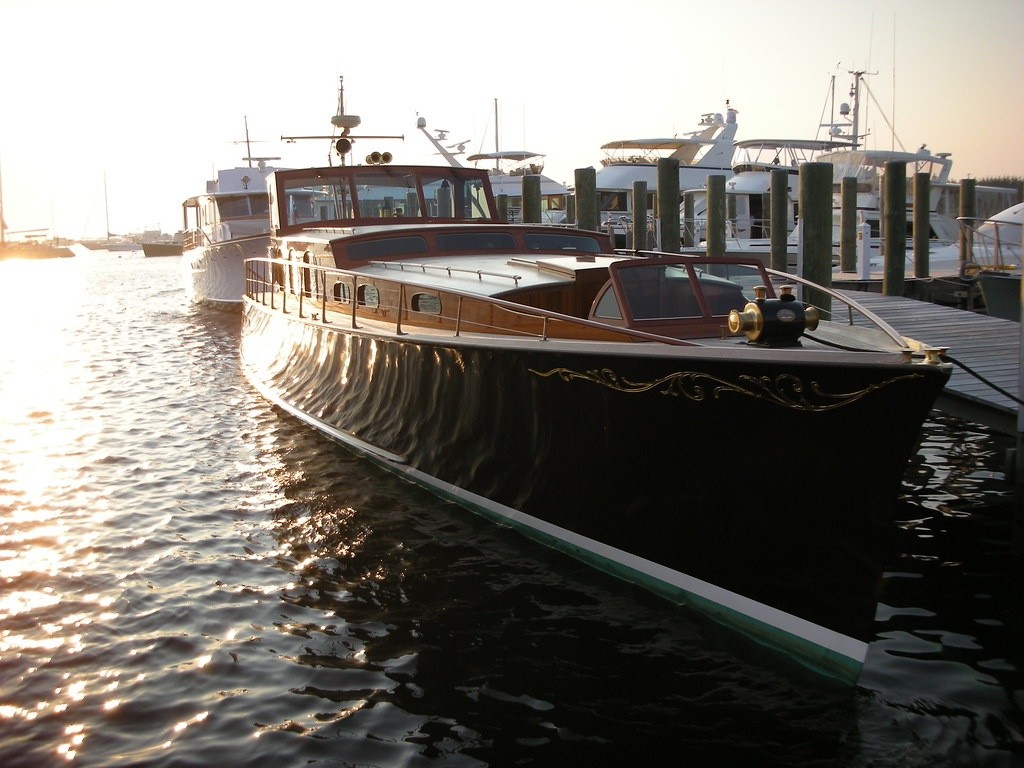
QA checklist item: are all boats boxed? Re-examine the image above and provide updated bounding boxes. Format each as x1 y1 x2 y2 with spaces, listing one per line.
335 66 1024 278
80 239 106 251
0 168 76 258
181 116 328 315
106 242 143 252
234 72 958 687
141 242 188 257
